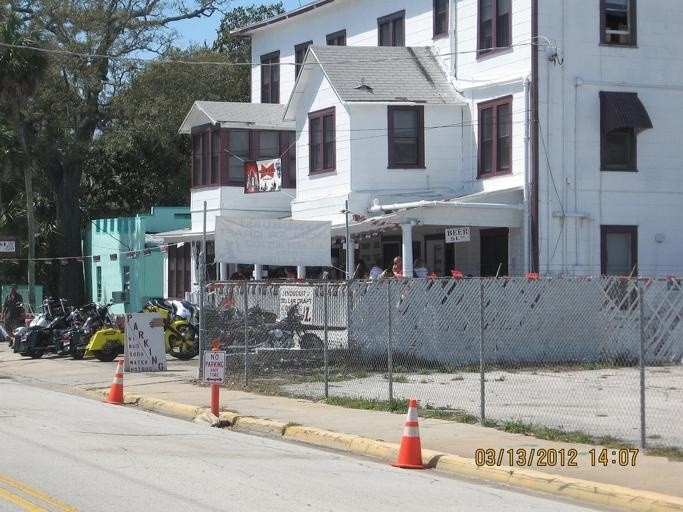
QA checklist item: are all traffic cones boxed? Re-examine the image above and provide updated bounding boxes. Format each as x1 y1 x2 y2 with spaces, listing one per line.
102 360 124 405
388 400 437 469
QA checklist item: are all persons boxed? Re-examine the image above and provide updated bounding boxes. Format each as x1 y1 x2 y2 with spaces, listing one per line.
1 283 25 348
227 256 427 279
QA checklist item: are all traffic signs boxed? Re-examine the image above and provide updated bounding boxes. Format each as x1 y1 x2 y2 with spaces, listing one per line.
202 350 226 384
